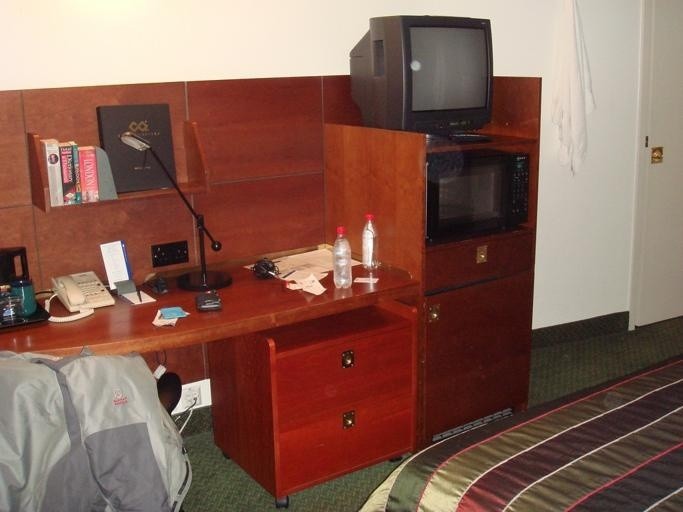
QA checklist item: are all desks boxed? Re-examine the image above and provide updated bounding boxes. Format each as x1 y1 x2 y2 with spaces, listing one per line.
1 249 417 362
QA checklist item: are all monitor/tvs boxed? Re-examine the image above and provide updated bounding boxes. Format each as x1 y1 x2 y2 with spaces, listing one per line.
428 151 532 243
350 15 494 131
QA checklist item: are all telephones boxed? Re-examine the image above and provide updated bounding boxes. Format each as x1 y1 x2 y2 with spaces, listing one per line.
44 271 117 322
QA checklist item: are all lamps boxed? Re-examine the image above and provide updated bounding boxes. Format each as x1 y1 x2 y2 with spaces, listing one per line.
120 132 234 292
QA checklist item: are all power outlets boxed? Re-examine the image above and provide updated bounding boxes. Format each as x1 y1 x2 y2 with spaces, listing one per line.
183 388 201 408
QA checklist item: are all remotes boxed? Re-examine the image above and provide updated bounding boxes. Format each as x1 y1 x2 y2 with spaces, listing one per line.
450 134 492 142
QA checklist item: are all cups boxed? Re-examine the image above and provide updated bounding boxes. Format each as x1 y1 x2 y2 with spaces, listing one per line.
12 281 37 318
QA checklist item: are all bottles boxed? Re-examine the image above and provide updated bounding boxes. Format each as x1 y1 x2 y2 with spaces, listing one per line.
362 214 379 271
333 226 353 289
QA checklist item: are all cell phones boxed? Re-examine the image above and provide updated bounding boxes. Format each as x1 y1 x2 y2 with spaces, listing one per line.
197 294 221 311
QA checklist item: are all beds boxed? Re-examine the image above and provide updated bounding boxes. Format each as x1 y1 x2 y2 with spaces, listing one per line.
360 351 683 512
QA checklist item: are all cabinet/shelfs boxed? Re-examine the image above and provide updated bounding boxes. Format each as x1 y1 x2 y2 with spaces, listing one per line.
211 301 419 508
27 122 214 213
323 125 538 457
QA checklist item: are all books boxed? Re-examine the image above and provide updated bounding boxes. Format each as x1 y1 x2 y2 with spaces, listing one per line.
99 240 132 291
40 138 99 207
97 103 178 193
